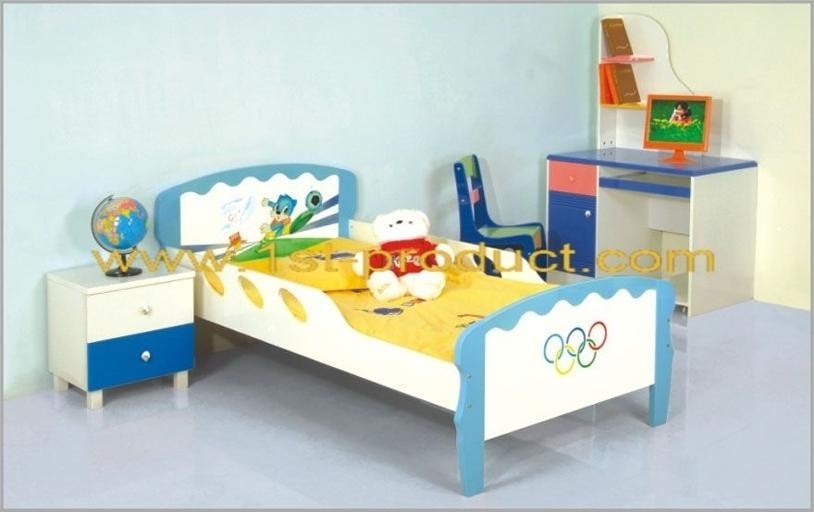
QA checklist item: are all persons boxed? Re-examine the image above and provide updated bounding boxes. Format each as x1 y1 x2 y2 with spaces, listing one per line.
668 102 692 124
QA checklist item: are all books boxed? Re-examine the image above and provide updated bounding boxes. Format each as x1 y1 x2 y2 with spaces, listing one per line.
599 17 643 105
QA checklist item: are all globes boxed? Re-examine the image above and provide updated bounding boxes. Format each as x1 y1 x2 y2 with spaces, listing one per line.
91 194 150 277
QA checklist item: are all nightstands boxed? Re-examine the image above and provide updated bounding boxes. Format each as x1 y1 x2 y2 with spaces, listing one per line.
45 258 195 407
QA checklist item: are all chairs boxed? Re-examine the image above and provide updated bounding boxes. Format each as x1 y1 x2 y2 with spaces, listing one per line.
455 155 547 287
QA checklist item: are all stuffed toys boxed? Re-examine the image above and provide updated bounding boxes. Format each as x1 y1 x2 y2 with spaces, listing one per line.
351 207 456 301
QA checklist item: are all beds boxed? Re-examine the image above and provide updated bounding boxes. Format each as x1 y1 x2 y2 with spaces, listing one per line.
154 164 676 496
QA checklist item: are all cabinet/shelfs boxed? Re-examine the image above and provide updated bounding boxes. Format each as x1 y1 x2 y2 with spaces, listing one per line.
597 16 654 149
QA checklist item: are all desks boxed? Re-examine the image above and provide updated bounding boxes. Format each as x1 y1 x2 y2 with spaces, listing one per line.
546 147 757 319
